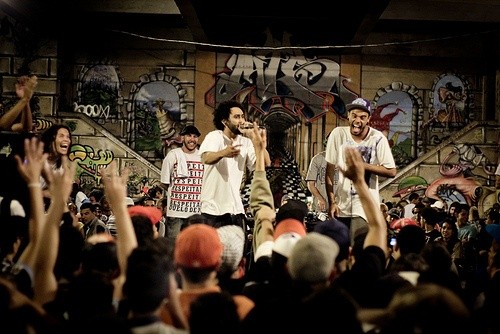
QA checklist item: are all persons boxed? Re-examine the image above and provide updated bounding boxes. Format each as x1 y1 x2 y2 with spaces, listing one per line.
0 137 500 334
306 132 332 213
325 97 397 247
197 101 271 228
39 124 73 198
0 75 38 131
161 125 204 238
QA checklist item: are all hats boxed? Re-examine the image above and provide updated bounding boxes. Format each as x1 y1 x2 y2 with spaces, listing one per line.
387 208 401 218
180 125 202 137
127 205 162 226
313 218 352 249
271 233 302 259
273 219 307 241
212 225 246 273
345 97 371 114
176 224 224 270
287 231 339 282
274 200 309 220
124 197 134 207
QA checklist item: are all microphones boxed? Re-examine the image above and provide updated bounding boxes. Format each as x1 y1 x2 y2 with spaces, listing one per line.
240 124 265 129
